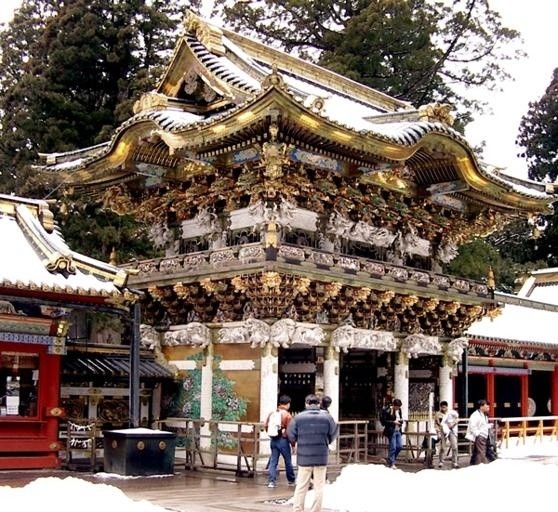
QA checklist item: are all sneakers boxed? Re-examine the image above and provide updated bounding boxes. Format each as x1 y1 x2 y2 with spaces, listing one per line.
390 465 397 470
451 462 460 468
287 480 296 486
438 462 443 467
268 481 279 488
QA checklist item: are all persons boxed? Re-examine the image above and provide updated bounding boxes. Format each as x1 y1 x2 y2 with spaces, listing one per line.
464 399 488 465
419 431 441 469
379 399 403 469
484 402 499 462
319 396 332 410
434 401 460 468
287 394 337 512
263 394 296 488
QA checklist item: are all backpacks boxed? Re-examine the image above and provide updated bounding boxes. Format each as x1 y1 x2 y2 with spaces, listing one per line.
267 409 285 437
